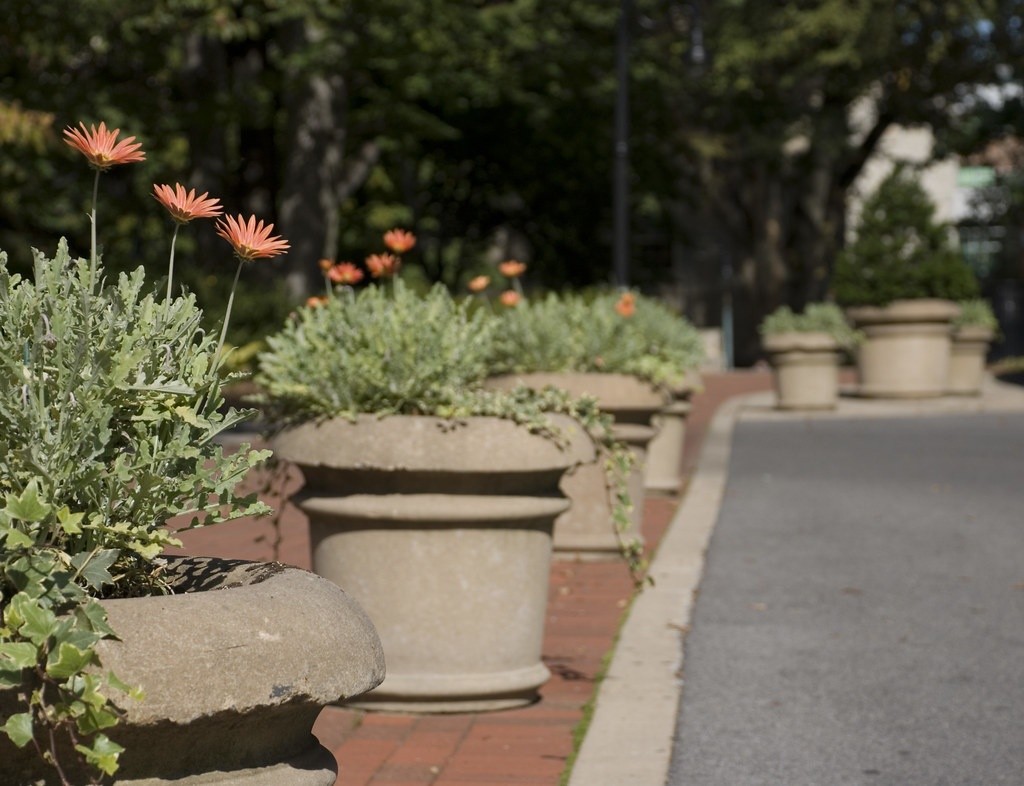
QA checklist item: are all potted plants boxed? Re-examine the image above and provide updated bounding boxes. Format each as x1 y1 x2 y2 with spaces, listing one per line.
838 163 974 403
758 302 865 410
946 299 998 405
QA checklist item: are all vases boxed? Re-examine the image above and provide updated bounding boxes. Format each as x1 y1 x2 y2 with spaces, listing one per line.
265 414 598 714
646 374 704 496
482 373 666 553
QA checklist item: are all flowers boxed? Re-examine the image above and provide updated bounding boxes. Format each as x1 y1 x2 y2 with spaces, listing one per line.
256 227 693 593
0 118 291 786
56 555 386 786
